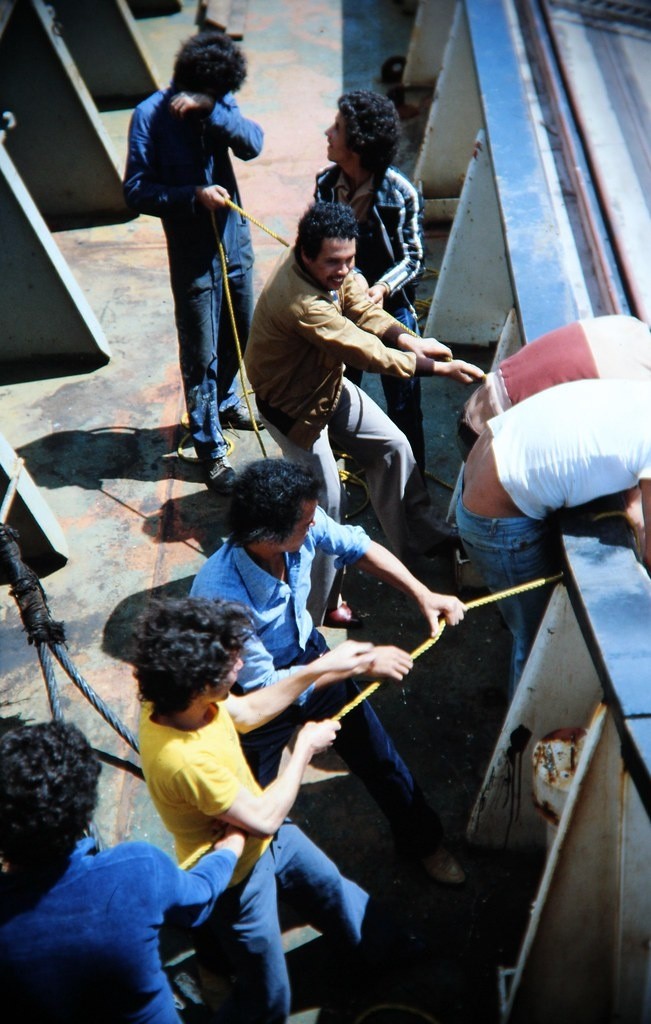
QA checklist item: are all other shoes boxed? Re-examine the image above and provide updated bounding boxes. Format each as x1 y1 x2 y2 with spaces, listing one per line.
221 404 262 430
359 935 427 970
199 446 240 496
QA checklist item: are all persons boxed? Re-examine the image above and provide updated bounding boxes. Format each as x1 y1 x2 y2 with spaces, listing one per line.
123 32 264 497
445 379 651 698
314 90 428 489
187 456 468 883
132 598 375 1024
243 202 488 626
0 719 244 1024
457 314 651 463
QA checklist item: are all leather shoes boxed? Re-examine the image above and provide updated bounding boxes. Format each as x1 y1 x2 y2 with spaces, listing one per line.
325 598 361 628
421 843 468 885
